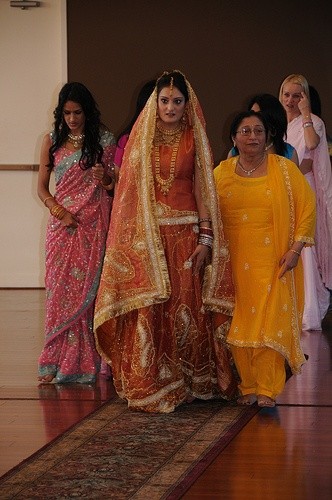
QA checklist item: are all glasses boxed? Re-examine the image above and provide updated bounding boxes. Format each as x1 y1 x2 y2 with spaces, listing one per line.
236 126 265 137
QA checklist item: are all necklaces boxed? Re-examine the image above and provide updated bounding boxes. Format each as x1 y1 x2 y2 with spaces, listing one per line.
265 141 274 151
154 120 185 195
67 133 85 148
237 152 266 176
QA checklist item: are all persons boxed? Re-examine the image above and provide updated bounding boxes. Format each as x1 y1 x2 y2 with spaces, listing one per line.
91 70 237 415
36 82 117 385
227 94 299 167
214 110 317 409
114 78 158 177
280 76 332 332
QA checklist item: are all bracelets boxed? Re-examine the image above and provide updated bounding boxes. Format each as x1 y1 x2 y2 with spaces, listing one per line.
198 227 213 249
290 249 301 256
103 181 114 190
44 197 67 220
303 121 313 128
301 115 311 119
199 218 212 223
